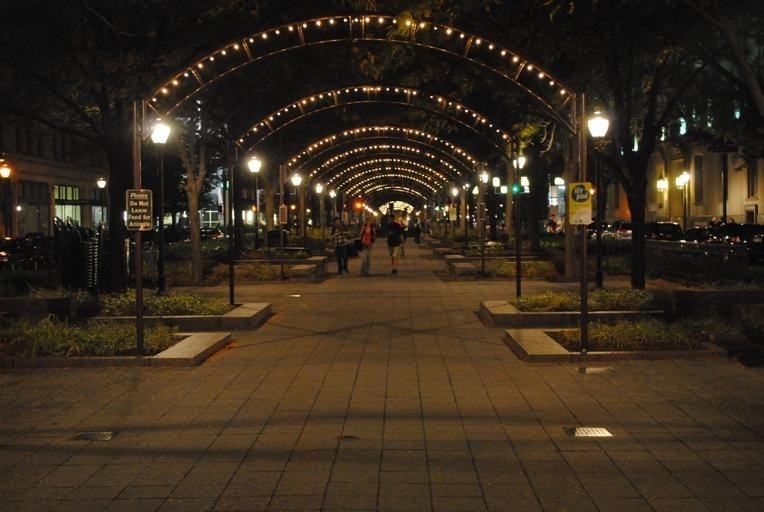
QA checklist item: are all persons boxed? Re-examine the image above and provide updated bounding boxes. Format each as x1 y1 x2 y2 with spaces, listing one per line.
413 216 423 244
358 217 378 276
547 215 558 230
401 214 408 242
328 216 351 274
386 214 403 275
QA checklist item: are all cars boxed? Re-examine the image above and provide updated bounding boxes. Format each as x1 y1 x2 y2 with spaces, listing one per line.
579 221 763 264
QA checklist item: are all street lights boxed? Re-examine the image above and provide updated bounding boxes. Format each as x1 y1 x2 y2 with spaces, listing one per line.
291 173 303 237
492 156 530 298
150 119 171 297
314 183 324 227
248 155 262 252
587 104 610 289
329 190 335 229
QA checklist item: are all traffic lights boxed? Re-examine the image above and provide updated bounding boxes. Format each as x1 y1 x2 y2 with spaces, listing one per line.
511 183 520 196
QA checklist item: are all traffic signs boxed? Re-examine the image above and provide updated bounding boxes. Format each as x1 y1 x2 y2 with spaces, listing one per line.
126 189 154 232
